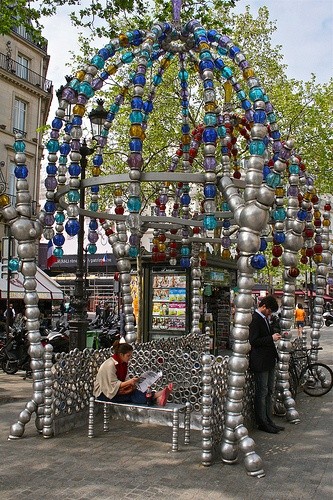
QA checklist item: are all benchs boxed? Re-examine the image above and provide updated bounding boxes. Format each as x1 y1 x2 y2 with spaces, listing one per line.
88 397 191 453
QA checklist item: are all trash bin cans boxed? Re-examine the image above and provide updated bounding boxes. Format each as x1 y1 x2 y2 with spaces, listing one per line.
87 328 103 349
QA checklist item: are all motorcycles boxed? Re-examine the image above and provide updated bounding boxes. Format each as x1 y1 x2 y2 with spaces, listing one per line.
0 311 126 379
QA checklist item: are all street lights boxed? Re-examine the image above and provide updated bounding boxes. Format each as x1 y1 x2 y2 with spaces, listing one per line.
56 74 110 353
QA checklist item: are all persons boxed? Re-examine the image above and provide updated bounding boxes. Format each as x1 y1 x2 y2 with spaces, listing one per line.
249 296 285 434
295 304 306 338
4 304 15 326
93 341 146 406
96 301 102 317
60 299 66 315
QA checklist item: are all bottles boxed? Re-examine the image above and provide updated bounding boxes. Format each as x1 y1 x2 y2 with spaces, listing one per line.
146 387 153 407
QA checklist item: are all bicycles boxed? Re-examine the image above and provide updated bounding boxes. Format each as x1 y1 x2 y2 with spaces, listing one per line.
288 347 333 401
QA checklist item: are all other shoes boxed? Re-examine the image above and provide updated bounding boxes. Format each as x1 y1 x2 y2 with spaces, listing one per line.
159 387 169 406
270 422 285 430
258 424 279 434
168 383 173 392
